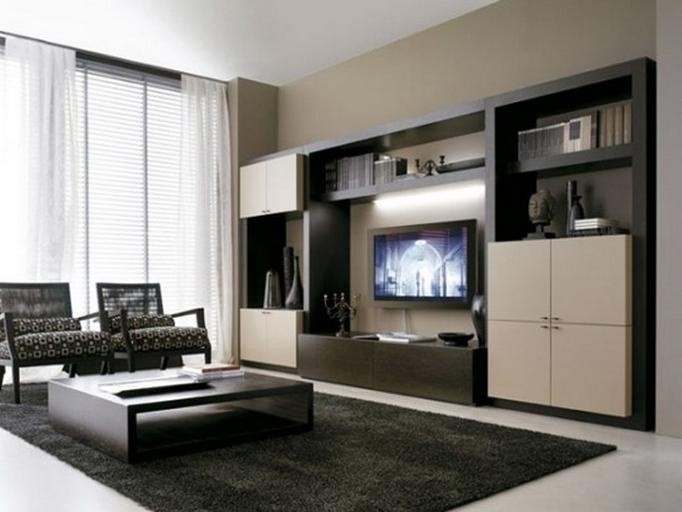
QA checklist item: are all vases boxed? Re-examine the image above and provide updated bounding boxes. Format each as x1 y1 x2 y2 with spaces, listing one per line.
282 255 304 309
471 293 487 346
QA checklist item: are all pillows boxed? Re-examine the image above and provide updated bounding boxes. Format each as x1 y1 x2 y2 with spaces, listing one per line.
111 314 176 334
2 314 79 338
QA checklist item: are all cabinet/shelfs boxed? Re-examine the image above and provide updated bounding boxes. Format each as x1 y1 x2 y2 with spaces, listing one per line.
486 233 653 432
300 332 487 406
483 56 656 240
239 306 350 376
239 139 351 223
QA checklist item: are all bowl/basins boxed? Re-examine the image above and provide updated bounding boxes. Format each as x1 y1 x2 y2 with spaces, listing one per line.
438 332 473 347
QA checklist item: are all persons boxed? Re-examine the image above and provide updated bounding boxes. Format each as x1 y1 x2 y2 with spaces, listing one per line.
527 187 558 225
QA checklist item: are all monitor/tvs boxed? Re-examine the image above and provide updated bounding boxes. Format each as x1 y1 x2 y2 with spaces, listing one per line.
367 217 477 308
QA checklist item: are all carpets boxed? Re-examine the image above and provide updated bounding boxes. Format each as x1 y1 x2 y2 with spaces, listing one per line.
1 380 620 512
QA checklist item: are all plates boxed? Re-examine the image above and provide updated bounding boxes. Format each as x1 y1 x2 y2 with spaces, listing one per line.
573 217 617 229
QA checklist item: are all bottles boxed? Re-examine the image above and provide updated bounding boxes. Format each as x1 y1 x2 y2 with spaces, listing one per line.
262 246 303 308
563 180 584 235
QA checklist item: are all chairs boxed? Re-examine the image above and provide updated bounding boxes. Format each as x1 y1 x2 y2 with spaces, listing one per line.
0 281 117 406
94 282 212 374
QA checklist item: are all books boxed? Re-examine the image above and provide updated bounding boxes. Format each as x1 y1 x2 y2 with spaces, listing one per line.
97 371 210 396
177 361 245 380
571 217 618 236
322 152 421 192
375 331 437 343
516 102 630 161
